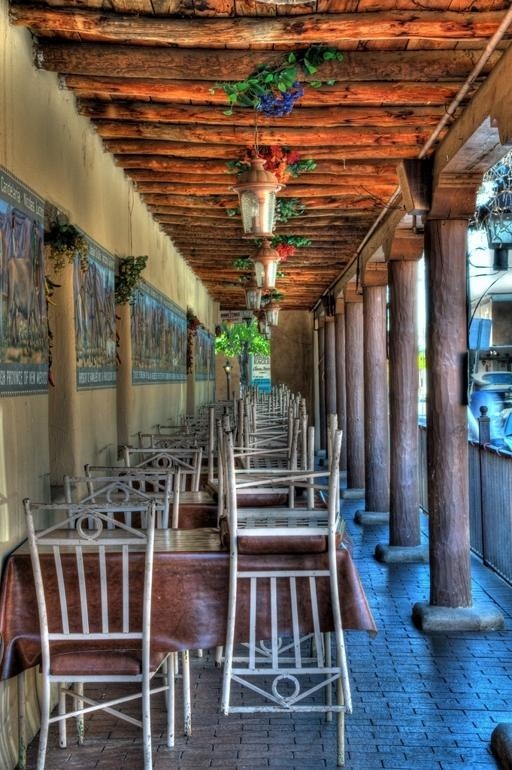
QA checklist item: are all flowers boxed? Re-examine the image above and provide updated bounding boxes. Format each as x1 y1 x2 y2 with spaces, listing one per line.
222 144 317 216
255 289 284 307
210 48 340 117
245 235 311 265
187 309 205 375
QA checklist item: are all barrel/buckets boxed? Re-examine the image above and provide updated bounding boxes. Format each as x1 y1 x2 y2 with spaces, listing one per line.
468 392 503 448
469 318 492 352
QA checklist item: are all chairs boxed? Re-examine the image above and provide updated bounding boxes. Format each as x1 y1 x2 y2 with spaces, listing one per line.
19 498 178 770
62 475 174 531
216 418 355 714
84 465 181 531
207 408 315 511
122 381 309 471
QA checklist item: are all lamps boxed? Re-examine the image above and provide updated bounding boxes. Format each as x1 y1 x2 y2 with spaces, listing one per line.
223 360 233 399
265 303 286 329
238 157 279 241
248 245 282 294
258 318 269 334
